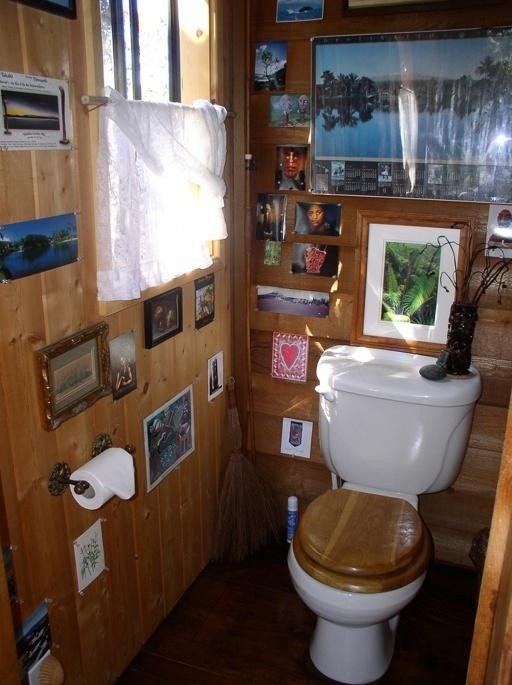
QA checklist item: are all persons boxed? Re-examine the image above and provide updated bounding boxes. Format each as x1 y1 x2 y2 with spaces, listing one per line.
279 147 304 191
487 209 512 251
304 202 334 236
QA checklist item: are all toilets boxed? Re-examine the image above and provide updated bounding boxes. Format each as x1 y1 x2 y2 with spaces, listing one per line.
287 345 483 685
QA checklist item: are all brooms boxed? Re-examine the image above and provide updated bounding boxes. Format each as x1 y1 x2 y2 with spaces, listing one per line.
213 375 282 565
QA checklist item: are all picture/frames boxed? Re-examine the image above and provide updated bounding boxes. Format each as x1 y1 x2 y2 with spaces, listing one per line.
347 206 476 358
33 319 115 432
141 284 184 348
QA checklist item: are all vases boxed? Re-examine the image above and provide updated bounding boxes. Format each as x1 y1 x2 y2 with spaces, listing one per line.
445 300 477 371
